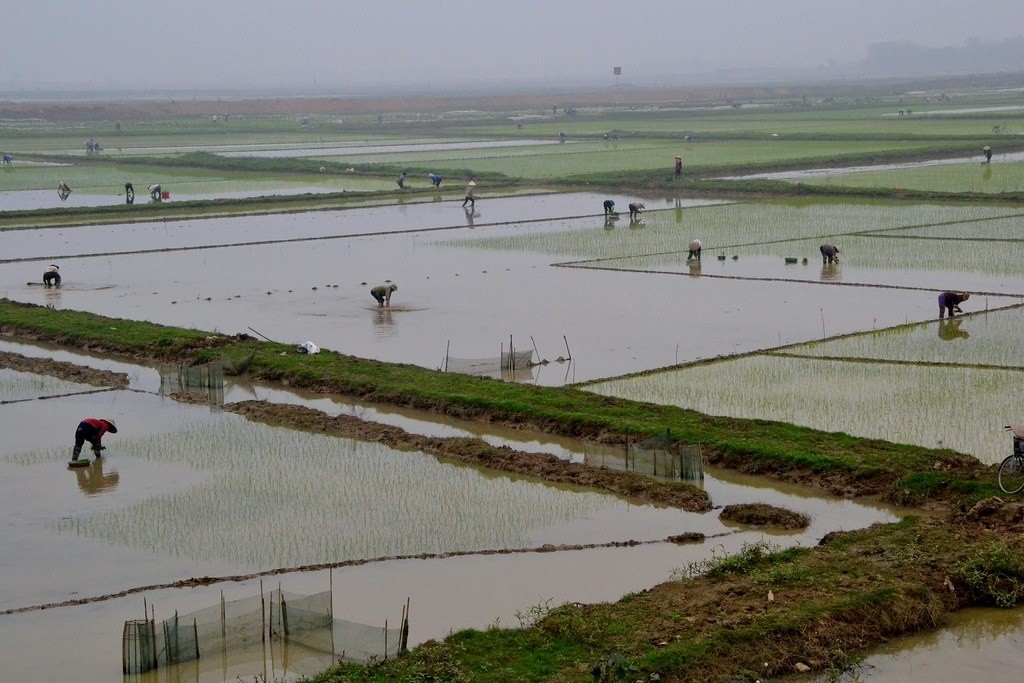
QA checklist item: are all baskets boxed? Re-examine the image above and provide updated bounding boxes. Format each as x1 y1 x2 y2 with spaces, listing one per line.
1013 436 1024 453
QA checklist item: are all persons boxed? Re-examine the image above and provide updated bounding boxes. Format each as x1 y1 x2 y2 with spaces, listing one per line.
428 174 442 187
674 156 682 180
396 171 407 188
58 181 72 191
72 418 117 460
147 184 161 196
685 135 694 143
604 200 615 214
820 244 841 265
125 181 134 192
463 181 476 206
3 155 14 164
116 120 121 132
212 114 217 123
628 202 645 218
370 283 397 308
43 265 61 286
938 292 970 318
688 239 701 258
983 145 993 164
85 139 99 151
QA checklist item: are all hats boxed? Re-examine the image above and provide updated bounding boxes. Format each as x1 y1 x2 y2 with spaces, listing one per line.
468 181 476 186
689 240 701 251
639 203 646 209
391 284 398 291
836 246 840 253
1011 424 1024 440
100 418 118 434
674 157 682 161
